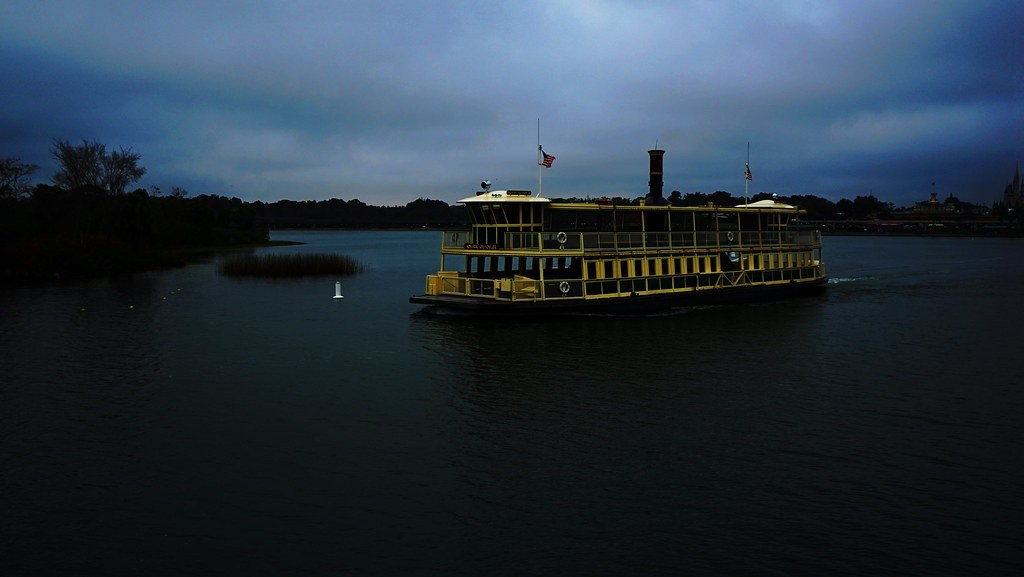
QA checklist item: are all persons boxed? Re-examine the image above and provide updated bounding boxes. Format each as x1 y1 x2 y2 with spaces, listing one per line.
548 263 576 273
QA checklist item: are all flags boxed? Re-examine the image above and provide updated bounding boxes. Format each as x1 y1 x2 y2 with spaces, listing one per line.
744 168 752 181
542 150 556 168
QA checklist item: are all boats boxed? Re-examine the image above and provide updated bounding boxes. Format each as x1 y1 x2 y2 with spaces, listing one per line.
409 114 827 314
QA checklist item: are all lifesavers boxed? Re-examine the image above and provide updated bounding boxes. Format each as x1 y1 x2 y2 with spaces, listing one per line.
451 232 459 242
727 232 734 240
559 281 570 293
557 232 567 243
